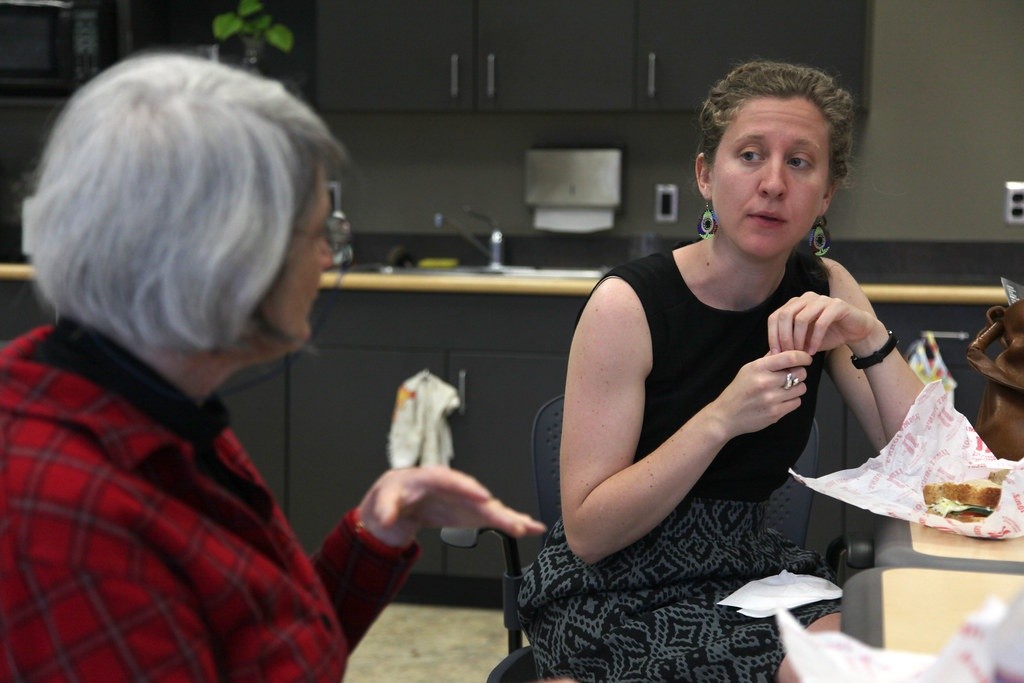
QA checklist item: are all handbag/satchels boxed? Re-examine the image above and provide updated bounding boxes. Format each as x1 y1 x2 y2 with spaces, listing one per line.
964 299 1024 461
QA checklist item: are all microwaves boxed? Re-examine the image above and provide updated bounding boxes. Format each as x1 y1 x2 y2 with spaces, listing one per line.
0 0 119 95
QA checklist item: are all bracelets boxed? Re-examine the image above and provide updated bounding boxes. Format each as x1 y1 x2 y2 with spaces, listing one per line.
353 510 411 556
851 331 899 369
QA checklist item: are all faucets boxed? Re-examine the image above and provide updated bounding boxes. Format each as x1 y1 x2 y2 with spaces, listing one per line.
432 210 503 274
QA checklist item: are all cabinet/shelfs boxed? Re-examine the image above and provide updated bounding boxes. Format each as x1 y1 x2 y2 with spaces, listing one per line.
0 277 1024 612
280 0 863 114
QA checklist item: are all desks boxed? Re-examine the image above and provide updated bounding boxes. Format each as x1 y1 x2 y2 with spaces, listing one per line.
834 453 1024 683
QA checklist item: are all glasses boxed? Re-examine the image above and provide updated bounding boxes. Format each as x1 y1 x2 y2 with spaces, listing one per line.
291 212 348 256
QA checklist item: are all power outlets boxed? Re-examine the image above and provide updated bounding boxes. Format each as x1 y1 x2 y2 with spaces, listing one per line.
329 182 341 216
1005 181 1024 225
654 184 678 221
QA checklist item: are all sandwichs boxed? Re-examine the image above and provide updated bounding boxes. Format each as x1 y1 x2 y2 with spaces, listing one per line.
922 479 1005 523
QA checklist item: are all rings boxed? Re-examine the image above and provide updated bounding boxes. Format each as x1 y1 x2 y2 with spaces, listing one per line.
783 369 798 389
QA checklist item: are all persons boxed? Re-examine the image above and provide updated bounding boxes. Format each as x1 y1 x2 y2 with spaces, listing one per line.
514 60 926 683
0 51 544 683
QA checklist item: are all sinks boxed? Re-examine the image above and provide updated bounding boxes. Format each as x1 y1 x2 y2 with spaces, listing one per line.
347 263 488 277
492 264 606 277
